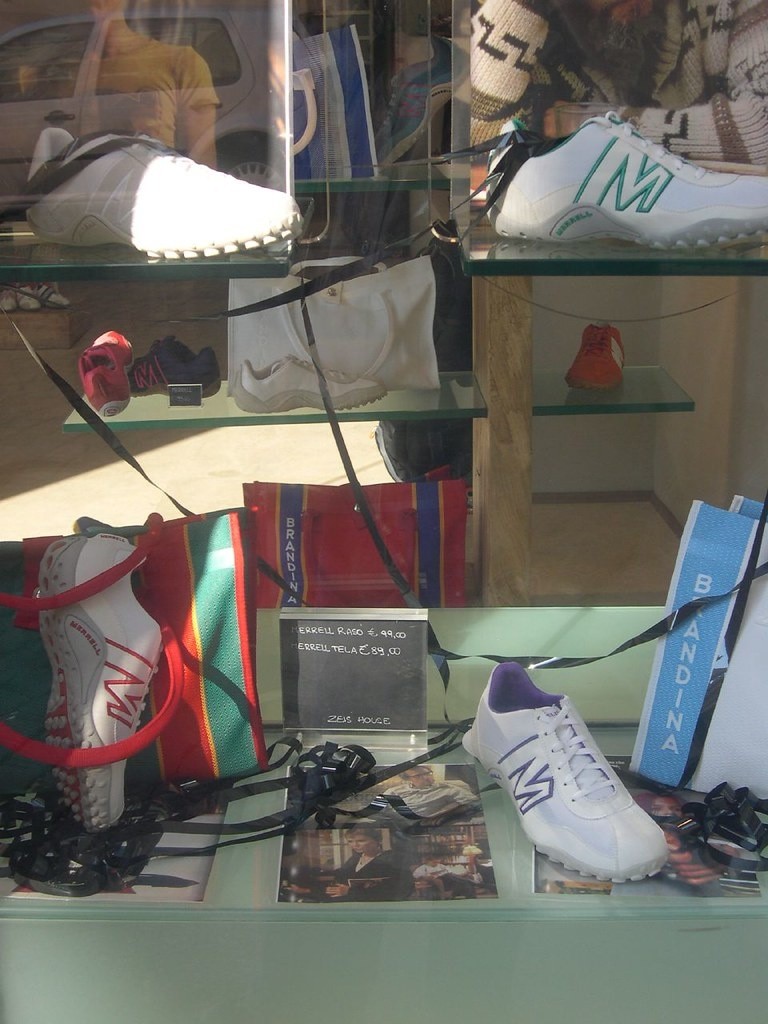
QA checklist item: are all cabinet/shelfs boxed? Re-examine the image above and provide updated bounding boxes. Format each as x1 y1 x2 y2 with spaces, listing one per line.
1 165 767 432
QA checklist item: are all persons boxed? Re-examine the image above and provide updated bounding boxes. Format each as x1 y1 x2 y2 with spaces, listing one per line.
469 0 768 194
412 852 476 899
377 765 480 827
461 844 492 883
287 826 416 903
17 1 220 176
609 790 740 897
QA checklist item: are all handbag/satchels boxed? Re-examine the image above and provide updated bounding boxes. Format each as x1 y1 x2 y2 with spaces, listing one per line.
0 503 270 797
290 21 376 178
245 465 473 607
625 494 768 802
226 258 441 395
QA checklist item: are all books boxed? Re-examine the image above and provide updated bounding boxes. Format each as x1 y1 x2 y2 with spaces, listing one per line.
696 833 762 896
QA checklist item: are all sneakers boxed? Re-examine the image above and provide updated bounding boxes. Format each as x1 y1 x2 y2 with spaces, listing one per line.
484 112 768 253
460 662 669 884
0 286 71 312
38 538 164 833
376 35 471 168
564 323 625 391
25 128 304 260
78 331 132 417
233 355 387 414
125 334 222 399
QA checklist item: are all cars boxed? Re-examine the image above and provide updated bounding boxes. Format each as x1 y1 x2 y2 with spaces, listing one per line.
0 7 316 215
